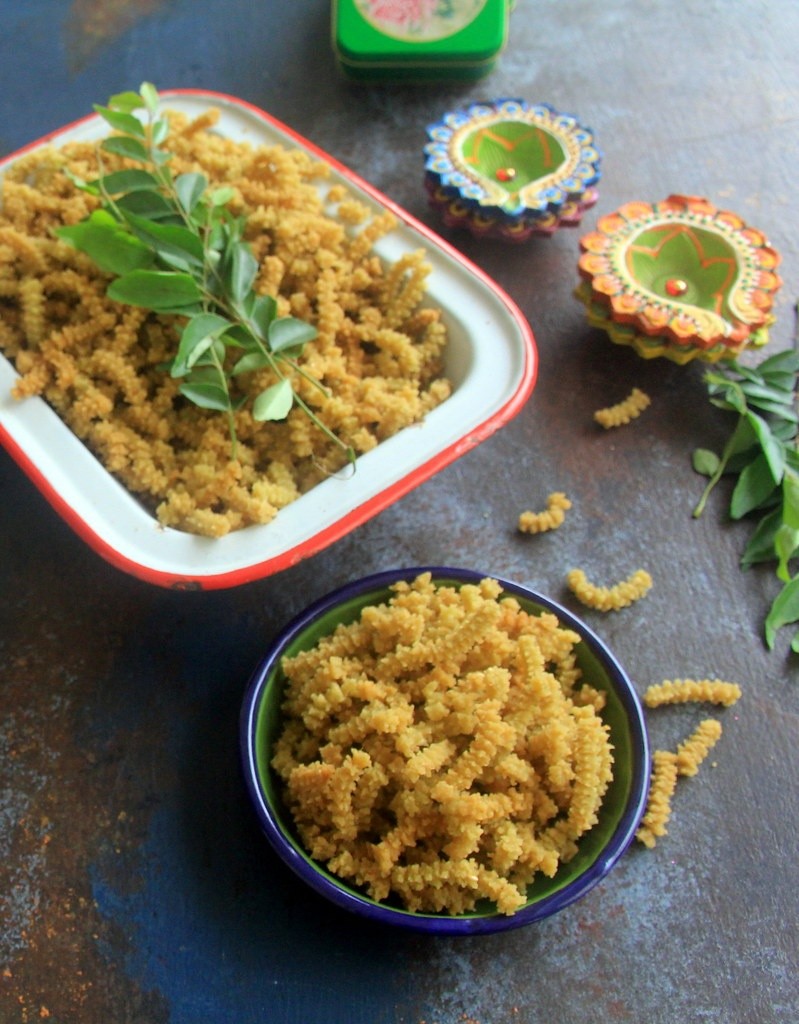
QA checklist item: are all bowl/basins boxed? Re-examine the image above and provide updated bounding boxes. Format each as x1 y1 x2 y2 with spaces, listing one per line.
0 86 540 593
241 564 652 934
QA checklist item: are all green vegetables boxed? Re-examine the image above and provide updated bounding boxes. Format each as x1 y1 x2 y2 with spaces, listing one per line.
688 345 799 658
60 85 354 465
